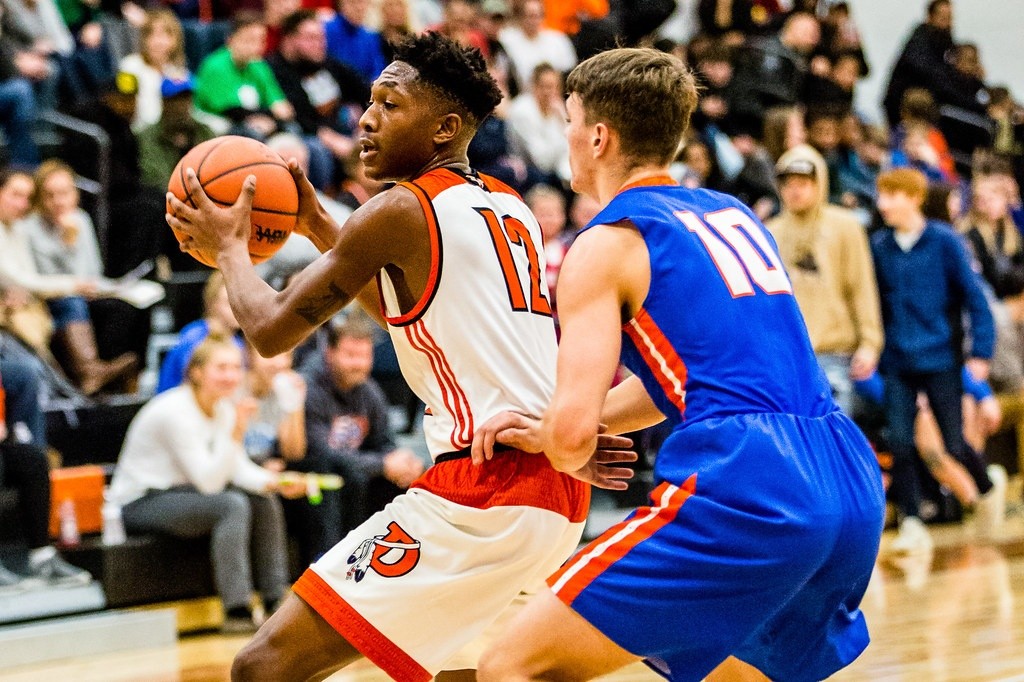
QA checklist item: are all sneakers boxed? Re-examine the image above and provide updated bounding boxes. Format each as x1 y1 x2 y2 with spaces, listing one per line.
0 545 91 591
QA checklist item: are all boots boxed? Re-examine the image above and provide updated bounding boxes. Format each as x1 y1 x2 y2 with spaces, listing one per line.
58 321 139 395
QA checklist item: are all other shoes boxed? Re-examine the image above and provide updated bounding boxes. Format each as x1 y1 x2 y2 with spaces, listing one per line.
897 514 929 547
221 609 276 634
977 463 1008 540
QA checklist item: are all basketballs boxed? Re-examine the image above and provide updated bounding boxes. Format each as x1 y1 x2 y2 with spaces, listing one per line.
165 135 299 269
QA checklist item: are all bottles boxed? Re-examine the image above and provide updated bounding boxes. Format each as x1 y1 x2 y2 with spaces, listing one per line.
60 500 79 544
99 491 127 544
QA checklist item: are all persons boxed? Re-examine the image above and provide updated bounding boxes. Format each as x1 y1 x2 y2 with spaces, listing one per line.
0 1 1024 682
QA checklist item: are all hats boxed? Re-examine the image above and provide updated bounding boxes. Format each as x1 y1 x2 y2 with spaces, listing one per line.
776 160 816 185
162 77 197 98
94 74 137 95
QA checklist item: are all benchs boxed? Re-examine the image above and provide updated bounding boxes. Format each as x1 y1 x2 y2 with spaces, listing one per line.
0 266 223 625
